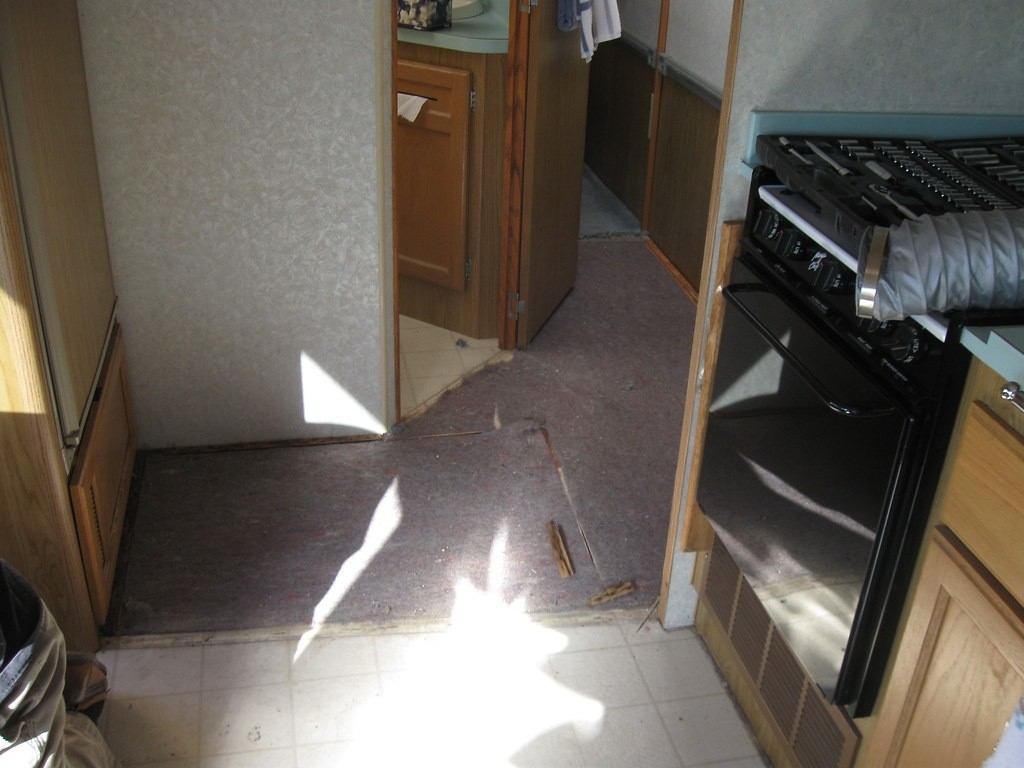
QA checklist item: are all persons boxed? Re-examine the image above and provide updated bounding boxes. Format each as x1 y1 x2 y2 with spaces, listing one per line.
1 557 124 767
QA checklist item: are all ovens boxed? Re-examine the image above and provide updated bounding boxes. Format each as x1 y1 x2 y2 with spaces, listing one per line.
690 253 960 719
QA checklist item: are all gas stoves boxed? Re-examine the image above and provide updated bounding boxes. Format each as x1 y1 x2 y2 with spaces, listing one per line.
743 131 1024 390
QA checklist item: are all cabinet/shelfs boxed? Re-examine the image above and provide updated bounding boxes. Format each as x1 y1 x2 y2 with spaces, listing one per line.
0 1 138 655
857 355 1024 768
395 40 507 342
